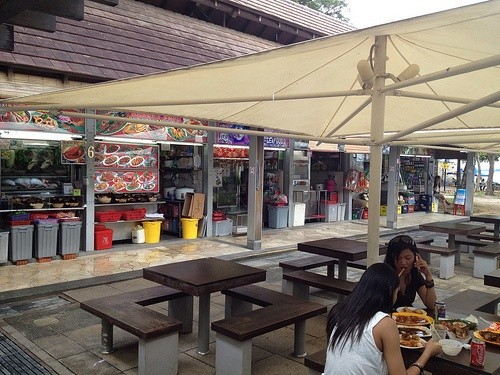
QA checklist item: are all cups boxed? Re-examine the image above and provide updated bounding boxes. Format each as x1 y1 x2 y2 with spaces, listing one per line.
430 324 448 343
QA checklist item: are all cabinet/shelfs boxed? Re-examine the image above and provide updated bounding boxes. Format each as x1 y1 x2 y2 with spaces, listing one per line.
159 144 198 172
160 199 184 238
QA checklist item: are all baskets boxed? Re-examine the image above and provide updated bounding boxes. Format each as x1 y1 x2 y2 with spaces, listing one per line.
47 213 75 218
61 218 81 222
35 218 59 225
119 208 146 221
11 220 32 226
30 213 49 219
94 225 105 231
10 214 29 221
95 210 123 222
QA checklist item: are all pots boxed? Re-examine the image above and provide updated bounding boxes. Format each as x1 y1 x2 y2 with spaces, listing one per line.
30 203 43 208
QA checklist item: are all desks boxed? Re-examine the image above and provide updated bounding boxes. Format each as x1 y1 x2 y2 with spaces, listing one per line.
470 216 500 237
297 237 388 302
229 211 249 237
143 256 267 355
419 222 486 248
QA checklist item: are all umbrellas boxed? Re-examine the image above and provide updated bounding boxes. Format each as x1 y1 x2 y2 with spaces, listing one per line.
0 0 499 271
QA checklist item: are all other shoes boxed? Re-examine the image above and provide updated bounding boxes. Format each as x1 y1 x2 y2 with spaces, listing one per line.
446 202 450 205
444 212 451 214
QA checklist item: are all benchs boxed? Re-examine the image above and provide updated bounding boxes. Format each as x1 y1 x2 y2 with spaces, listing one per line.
80 221 500 375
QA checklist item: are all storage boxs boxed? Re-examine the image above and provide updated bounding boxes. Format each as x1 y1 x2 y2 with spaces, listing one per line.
94 228 113 250
381 206 387 216
397 205 414 214
320 200 347 223
213 212 233 237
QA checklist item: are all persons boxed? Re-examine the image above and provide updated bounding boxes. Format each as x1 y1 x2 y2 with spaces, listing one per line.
480 177 486 191
321 263 442 375
385 234 436 311
433 176 451 214
453 178 457 186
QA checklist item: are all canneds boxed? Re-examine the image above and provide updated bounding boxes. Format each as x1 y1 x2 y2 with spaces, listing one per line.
469 339 485 367
434 301 446 320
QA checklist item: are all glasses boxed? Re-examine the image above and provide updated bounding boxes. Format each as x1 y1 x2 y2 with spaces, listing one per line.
390 237 415 249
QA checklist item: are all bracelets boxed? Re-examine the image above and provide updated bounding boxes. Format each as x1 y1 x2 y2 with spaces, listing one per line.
411 363 424 373
424 279 434 288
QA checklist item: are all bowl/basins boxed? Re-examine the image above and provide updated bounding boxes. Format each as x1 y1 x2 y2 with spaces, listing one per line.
53 203 63 207
98 198 111 203
438 339 464 356
2 110 32 128
149 198 157 201
65 202 79 206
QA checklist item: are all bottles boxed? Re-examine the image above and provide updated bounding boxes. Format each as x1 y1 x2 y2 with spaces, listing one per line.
434 301 446 324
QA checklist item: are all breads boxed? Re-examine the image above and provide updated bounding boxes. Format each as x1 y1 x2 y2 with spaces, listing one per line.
399 339 419 347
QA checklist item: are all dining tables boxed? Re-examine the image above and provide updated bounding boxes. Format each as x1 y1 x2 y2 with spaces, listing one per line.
402 311 500 375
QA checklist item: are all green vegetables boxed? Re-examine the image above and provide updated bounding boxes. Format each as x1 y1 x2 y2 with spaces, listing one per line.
440 317 477 330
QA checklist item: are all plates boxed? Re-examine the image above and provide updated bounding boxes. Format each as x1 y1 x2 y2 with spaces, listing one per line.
104 145 120 155
397 325 432 337
96 114 205 141
94 172 155 191
392 313 434 326
103 155 156 167
63 146 83 161
396 307 427 315
473 331 500 345
399 335 428 349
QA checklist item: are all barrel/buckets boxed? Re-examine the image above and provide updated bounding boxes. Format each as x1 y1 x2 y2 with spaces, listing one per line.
142 221 162 244
181 218 199 240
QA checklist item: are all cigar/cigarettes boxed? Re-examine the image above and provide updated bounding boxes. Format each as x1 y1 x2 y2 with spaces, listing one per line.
398 268 405 277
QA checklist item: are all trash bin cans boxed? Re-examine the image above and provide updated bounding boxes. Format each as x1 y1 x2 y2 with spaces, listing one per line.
59 221 82 255
321 204 338 222
8 225 34 262
180 217 199 240
266 205 289 229
1 229 10 264
36 218 59 259
142 221 162 243
